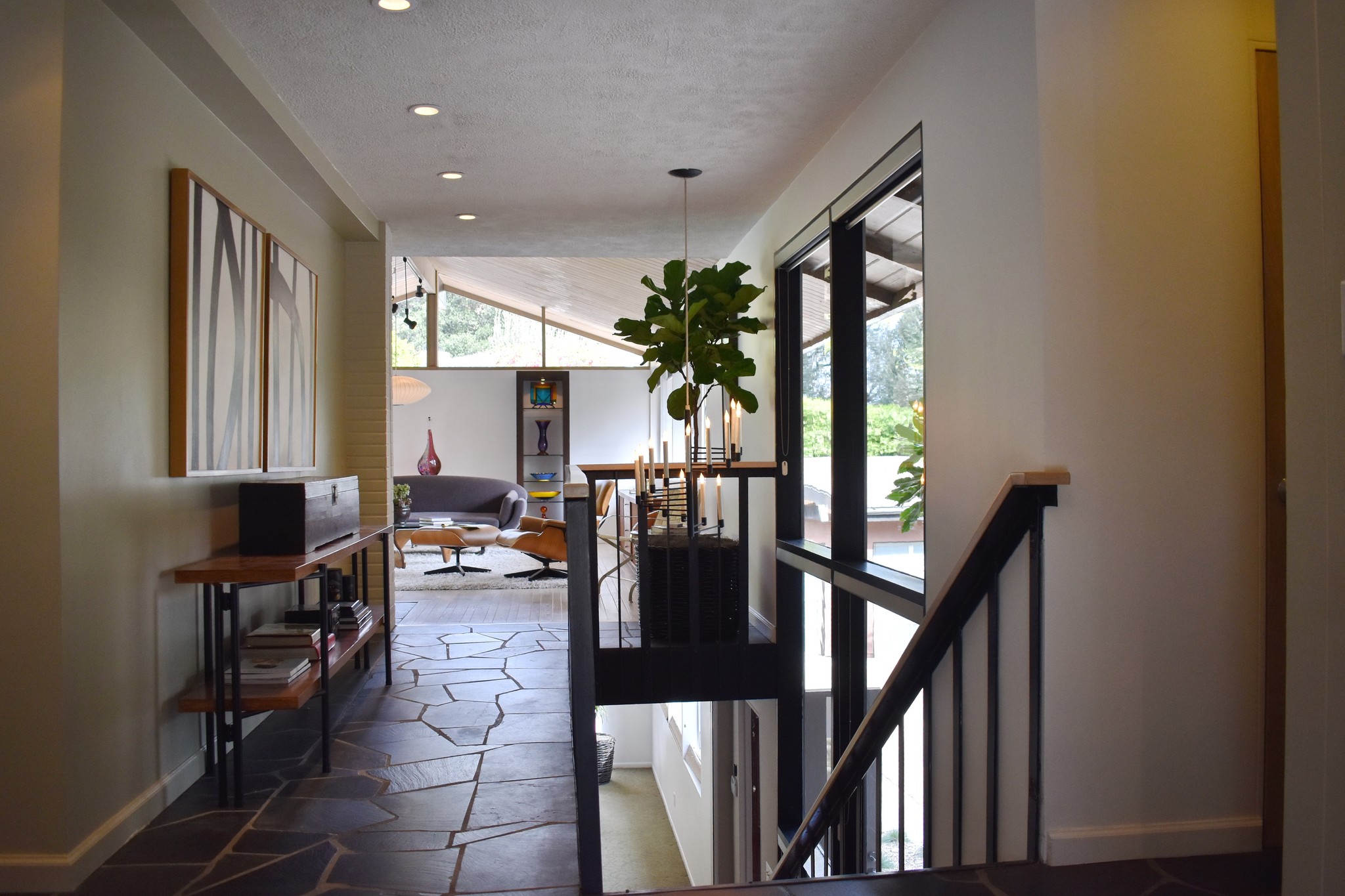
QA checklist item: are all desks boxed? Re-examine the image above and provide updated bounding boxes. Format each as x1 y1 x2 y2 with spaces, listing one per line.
394 523 424 569
175 525 394 802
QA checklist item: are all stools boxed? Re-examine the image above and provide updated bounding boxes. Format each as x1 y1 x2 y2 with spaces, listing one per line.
411 524 501 577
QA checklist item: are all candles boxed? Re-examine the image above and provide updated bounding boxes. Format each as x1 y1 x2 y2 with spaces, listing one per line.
705 416 712 465
638 444 645 492
699 473 706 518
731 399 744 453
648 480 653 503
685 423 691 473
663 474 667 509
648 437 655 485
663 430 669 478
680 469 687 514
725 410 731 459
716 474 723 520
634 449 641 496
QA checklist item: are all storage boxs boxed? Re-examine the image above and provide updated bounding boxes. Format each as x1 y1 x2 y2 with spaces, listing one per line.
239 475 359 555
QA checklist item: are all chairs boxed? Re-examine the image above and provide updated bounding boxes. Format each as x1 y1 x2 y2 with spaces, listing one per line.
495 480 616 582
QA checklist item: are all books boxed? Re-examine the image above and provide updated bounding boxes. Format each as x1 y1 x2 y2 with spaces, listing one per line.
245 622 336 661
420 516 454 526
224 652 311 685
651 522 701 536
337 605 373 631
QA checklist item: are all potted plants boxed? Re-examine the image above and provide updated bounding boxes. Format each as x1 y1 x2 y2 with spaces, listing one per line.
613 260 769 646
393 484 412 524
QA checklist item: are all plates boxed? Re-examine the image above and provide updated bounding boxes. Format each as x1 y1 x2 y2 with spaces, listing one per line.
530 381 557 406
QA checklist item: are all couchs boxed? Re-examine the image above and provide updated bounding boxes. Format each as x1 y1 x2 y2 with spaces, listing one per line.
393 476 528 531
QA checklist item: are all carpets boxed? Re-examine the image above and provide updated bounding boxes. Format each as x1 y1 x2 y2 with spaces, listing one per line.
394 544 568 591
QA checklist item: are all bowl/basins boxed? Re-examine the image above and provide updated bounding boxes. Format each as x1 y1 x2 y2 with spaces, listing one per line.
528 491 561 502
529 473 558 481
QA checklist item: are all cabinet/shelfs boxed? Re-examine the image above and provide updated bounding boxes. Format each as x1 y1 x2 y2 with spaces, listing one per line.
170 168 319 477
617 490 662 567
516 371 570 521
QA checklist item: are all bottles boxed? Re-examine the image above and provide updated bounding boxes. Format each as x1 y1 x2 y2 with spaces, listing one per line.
535 420 552 455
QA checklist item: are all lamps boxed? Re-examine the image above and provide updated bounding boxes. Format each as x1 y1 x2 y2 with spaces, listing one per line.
392 256 431 406
